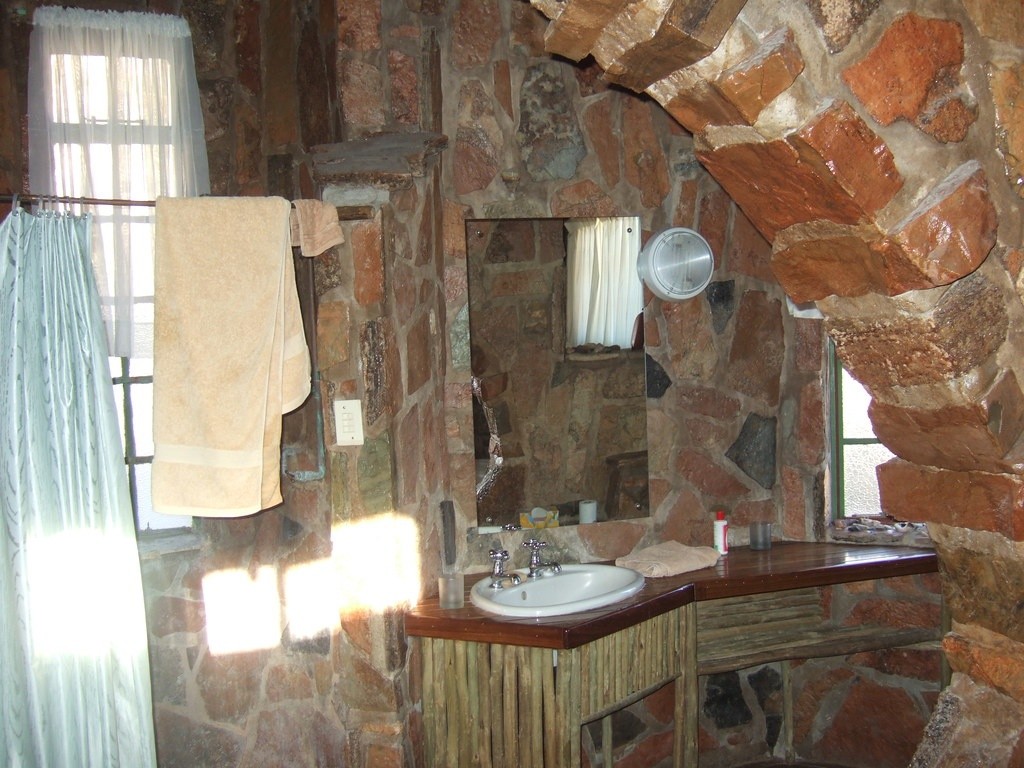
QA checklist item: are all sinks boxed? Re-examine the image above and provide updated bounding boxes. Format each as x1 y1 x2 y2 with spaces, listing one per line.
468 561 645 619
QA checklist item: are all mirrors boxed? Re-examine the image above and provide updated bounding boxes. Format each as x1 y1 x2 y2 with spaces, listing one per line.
463 214 650 534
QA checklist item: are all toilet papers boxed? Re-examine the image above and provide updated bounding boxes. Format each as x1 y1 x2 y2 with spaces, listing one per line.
577 498 598 525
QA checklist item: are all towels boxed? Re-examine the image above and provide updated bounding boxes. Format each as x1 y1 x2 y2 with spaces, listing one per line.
614 539 724 579
289 198 346 258
149 194 314 520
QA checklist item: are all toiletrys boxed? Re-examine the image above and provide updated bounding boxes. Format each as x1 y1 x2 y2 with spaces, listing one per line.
712 509 730 556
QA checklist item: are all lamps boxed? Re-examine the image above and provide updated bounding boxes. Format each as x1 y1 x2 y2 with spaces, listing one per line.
641 229 714 304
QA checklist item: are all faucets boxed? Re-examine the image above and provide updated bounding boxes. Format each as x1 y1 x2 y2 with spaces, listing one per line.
487 547 523 591
520 537 565 580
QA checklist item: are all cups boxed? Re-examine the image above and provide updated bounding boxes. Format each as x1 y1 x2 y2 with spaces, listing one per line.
438 573 465 609
749 521 772 552
